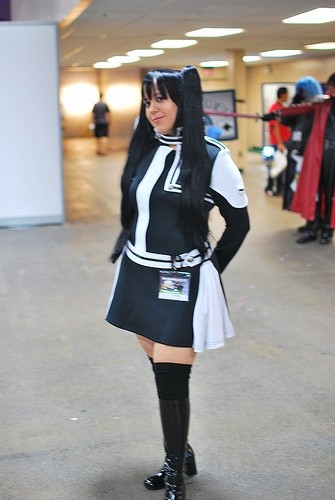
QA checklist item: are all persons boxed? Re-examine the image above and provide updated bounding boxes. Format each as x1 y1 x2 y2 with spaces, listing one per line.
264 74 335 246
106 67 249 500
90 93 110 156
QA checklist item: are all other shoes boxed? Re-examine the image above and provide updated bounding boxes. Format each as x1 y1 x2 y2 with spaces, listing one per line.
319 231 331 243
296 232 318 243
298 220 314 233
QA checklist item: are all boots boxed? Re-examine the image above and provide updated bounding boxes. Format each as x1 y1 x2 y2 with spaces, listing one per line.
144 357 197 499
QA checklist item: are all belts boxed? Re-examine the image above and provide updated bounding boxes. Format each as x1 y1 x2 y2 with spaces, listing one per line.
123 239 214 270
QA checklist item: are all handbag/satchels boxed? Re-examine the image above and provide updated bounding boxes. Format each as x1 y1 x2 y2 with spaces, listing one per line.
270 147 288 178
